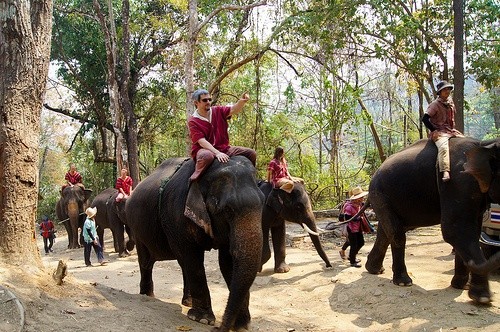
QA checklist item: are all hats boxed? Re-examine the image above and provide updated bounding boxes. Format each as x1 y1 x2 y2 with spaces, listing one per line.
435 80 453 95
85 206 98 218
349 187 368 199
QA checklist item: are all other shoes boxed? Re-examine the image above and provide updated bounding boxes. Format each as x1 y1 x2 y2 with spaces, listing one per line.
87 264 93 267
339 251 345 259
352 263 361 267
354 259 360 262
100 261 109 265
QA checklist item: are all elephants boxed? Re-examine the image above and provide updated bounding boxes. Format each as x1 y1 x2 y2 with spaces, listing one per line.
124 154 266 329
87 189 134 258
256 175 333 272
55 182 93 250
325 133 499 306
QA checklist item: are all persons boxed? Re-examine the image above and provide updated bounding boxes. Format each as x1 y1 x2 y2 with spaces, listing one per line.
188 90 256 180
83 207 109 267
115 169 132 202
339 190 361 261
62 165 85 192
264 146 304 193
345 186 374 268
422 81 464 180
39 217 54 254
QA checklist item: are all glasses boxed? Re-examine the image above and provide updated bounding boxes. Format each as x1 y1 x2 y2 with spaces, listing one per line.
199 97 212 102
